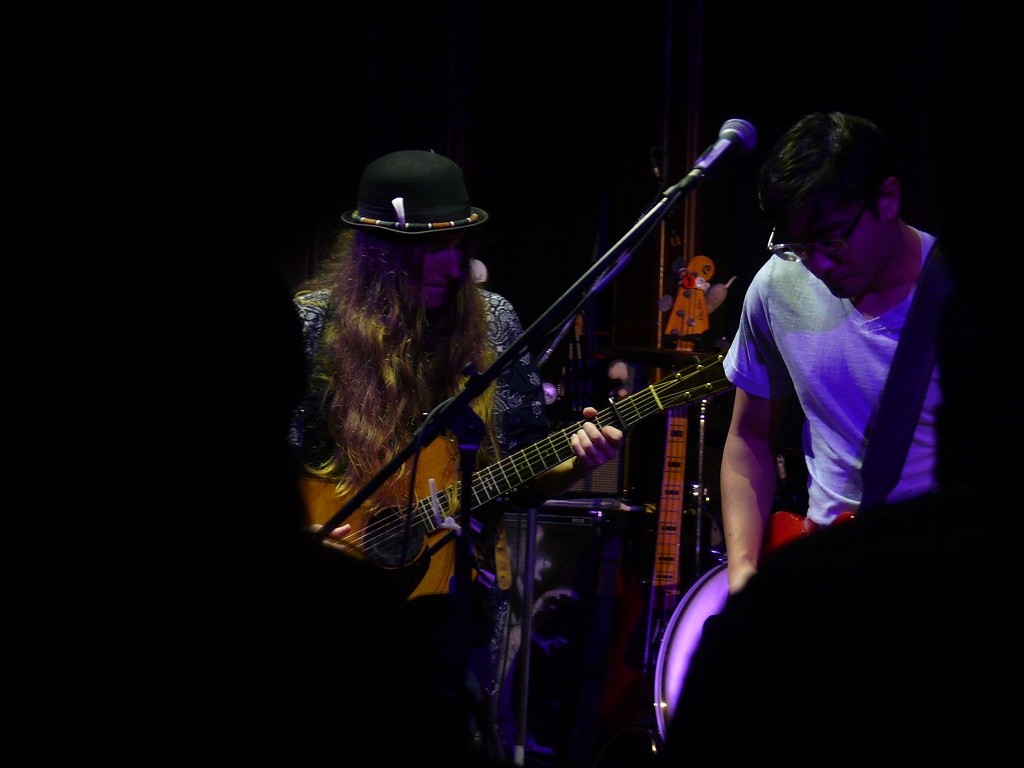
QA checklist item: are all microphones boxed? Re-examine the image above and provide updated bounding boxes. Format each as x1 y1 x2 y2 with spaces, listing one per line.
647 153 682 248
678 119 756 198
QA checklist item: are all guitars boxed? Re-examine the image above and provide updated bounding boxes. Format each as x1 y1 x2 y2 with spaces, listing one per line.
644 256 715 689
761 510 854 562
296 344 735 602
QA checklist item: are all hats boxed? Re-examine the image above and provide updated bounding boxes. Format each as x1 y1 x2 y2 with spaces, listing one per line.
339 151 489 236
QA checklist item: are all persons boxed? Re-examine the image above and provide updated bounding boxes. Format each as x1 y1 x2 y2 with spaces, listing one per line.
720 115 945 596
658 318 1024 768
254 150 624 768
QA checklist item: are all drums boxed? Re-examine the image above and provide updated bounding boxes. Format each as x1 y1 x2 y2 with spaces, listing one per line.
652 551 728 740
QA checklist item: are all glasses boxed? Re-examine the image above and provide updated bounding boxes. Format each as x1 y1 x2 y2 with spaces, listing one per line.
768 198 870 257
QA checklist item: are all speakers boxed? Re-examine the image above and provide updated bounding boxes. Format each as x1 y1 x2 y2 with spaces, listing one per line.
496 510 631 768
